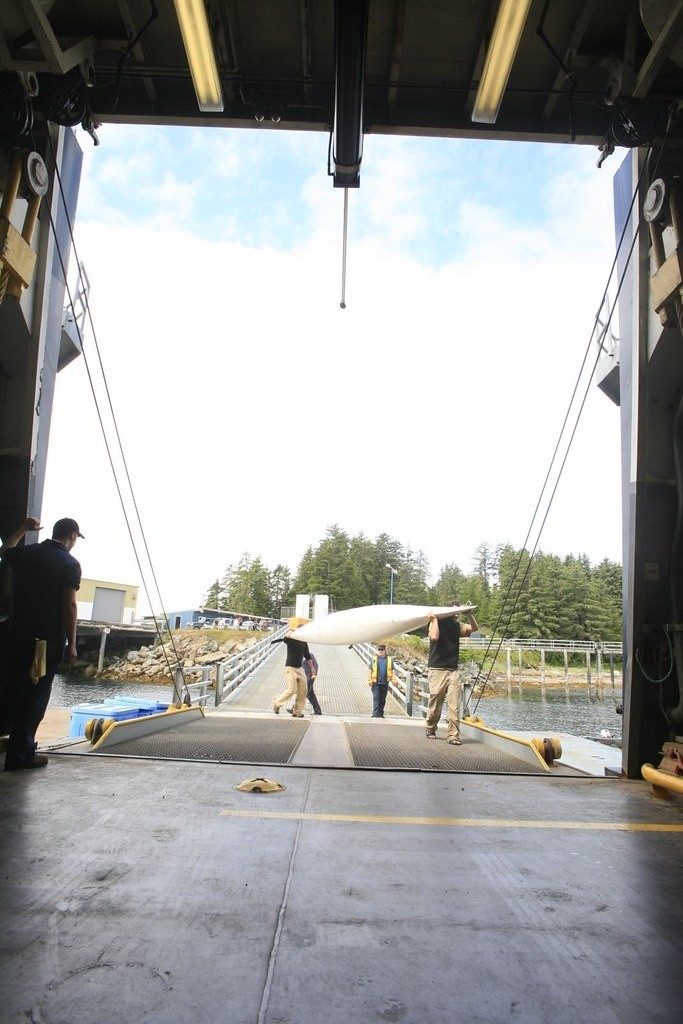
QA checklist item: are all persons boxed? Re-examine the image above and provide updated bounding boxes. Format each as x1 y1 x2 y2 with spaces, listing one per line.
424 601 479 745
272 624 322 717
236 615 286 631
367 645 394 718
1 517 86 770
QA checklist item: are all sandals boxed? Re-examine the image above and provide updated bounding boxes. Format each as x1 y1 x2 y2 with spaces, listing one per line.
426 730 436 739
450 739 461 745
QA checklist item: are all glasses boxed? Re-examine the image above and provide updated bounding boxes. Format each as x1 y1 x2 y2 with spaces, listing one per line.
378 649 383 651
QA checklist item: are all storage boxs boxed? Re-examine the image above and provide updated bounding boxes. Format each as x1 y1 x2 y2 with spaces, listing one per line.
70 696 171 738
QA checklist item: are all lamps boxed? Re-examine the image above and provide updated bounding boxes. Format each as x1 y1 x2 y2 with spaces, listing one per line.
172 0 226 116
466 0 531 124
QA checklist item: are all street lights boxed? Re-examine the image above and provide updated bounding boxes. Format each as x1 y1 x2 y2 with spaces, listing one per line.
385 563 399 604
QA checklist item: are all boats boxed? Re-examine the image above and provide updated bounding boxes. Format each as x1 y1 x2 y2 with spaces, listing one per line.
289 603 479 646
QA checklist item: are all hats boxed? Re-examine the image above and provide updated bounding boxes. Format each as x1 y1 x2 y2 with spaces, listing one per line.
53 518 85 540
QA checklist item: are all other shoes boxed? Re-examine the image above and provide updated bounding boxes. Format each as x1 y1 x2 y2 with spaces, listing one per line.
310 711 322 715
5 753 49 769
371 714 379 718
272 702 280 714
380 714 384 718
286 707 293 714
292 713 304 718
